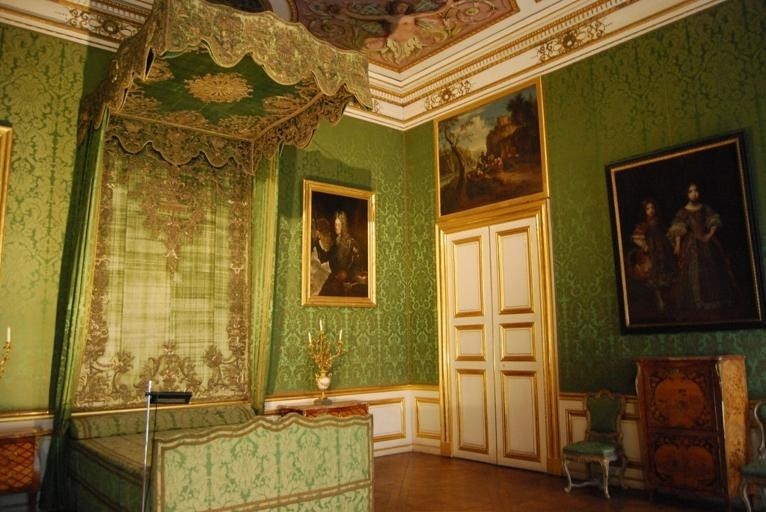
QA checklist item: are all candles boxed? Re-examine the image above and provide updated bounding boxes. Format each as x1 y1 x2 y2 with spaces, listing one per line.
308 319 343 345
6 325 11 343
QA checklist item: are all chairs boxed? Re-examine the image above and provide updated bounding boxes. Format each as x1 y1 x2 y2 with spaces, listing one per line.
738 399 766 512
562 389 630 499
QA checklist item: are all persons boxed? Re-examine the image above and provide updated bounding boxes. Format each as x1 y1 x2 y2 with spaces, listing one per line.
664 181 734 306
476 150 504 182
627 196 672 311
344 1 455 66
314 210 362 296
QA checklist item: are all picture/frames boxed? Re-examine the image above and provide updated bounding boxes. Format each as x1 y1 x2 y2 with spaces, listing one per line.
604 132 766 336
300 177 379 309
432 74 550 222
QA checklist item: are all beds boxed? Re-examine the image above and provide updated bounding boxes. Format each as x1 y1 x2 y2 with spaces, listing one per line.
65 402 376 512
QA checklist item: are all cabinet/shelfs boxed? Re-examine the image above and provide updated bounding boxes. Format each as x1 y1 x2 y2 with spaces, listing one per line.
277 400 369 419
626 354 750 509
0 427 42 512
